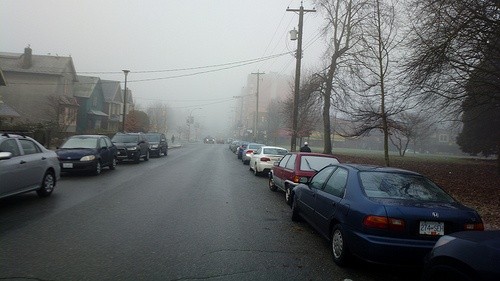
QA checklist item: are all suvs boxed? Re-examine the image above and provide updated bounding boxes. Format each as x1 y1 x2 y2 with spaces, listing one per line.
112 130 150 163
144 132 168 158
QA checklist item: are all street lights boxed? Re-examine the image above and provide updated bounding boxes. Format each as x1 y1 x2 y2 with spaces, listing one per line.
122 69 131 134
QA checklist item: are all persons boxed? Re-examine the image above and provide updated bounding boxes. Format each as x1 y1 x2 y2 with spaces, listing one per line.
300 142 312 153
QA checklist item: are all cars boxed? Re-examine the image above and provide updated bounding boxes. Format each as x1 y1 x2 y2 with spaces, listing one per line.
0 133 62 200
54 135 118 174
269 152 342 205
203 136 216 145
289 163 485 272
241 143 266 164
419 230 500 281
226 137 252 159
249 146 290 176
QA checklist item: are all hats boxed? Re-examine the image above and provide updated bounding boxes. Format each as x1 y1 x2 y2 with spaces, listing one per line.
304 142 308 145
